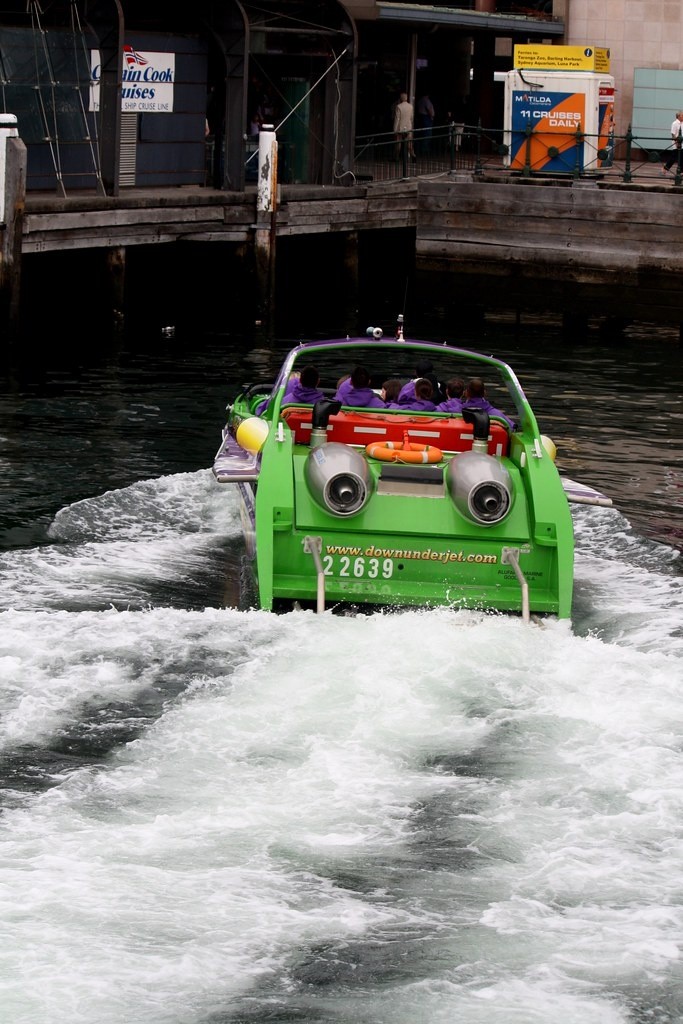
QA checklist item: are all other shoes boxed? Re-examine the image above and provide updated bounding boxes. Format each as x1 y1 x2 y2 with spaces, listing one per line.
680 173 683 176
663 167 667 174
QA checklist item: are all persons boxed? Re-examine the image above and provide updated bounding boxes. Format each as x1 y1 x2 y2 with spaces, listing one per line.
251 112 264 136
394 93 414 160
256 365 516 431
661 112 683 176
420 96 434 136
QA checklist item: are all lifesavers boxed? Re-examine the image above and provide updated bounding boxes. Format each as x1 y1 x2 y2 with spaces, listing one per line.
365 441 444 464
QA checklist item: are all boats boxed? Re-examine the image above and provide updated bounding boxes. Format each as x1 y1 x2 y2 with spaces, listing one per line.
225 315 575 622
